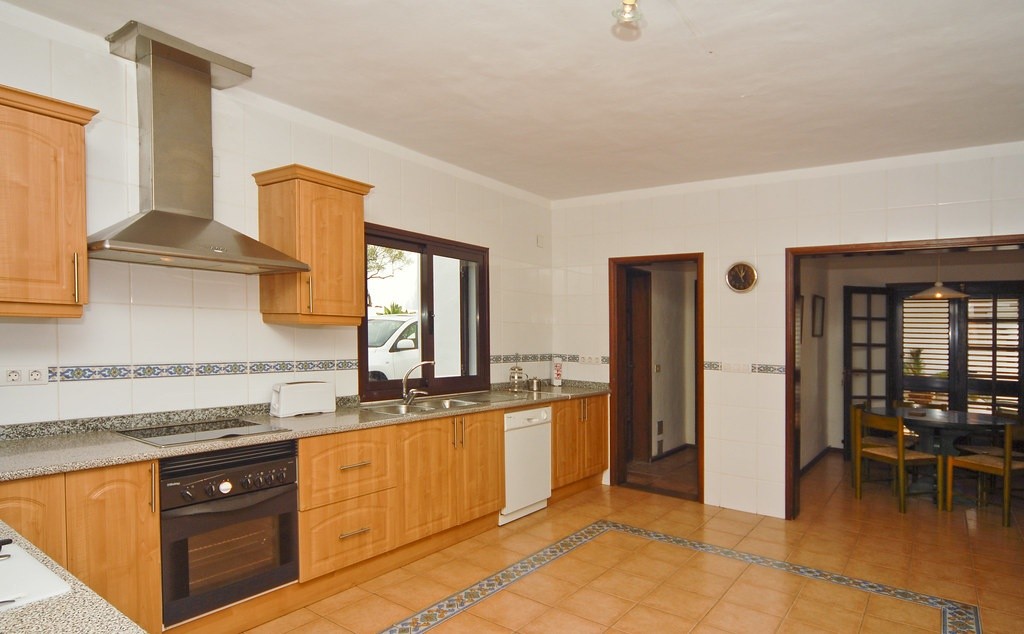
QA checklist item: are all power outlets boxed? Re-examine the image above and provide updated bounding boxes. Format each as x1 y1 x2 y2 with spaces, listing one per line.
0 367 49 385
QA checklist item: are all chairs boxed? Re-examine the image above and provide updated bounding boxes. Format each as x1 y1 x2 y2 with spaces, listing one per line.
849 399 1024 527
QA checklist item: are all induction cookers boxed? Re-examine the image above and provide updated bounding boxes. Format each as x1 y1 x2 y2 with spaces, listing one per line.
111 417 290 448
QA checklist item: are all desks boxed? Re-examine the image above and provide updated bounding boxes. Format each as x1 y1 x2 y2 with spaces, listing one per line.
864 407 1019 508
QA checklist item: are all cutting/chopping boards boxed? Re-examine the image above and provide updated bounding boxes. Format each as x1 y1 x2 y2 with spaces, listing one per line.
0 543 71 611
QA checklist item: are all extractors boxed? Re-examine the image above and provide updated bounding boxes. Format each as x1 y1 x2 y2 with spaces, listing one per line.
88 19 311 276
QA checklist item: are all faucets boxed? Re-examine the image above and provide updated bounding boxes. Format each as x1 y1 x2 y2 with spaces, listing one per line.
402 361 435 405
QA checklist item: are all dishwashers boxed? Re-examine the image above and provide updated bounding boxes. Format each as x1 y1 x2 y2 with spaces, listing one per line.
499 406 552 527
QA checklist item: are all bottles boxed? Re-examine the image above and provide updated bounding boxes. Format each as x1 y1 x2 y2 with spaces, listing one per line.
508 366 528 392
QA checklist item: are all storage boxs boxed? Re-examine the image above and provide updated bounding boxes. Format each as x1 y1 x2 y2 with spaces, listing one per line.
551 358 562 386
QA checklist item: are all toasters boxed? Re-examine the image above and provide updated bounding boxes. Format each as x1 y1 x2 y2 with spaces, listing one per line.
270 379 337 417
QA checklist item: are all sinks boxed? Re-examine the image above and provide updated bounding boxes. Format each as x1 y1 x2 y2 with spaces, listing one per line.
358 404 434 416
411 399 480 410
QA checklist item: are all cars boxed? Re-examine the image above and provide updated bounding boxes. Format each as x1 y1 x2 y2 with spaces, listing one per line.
368 313 419 383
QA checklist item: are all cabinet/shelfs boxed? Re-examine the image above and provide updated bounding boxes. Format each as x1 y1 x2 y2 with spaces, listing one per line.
251 163 377 327
399 409 507 567
296 424 404 610
0 85 100 318
0 460 162 634
548 394 608 505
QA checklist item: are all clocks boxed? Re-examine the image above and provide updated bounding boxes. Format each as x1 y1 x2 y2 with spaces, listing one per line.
725 262 758 293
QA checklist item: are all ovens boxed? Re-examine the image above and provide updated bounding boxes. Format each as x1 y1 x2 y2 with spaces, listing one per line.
160 458 299 629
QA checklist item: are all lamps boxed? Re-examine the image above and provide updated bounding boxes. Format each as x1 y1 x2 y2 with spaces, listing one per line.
612 0 643 22
911 249 971 299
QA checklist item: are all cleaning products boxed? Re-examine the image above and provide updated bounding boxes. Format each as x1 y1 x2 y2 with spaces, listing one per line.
552 358 562 386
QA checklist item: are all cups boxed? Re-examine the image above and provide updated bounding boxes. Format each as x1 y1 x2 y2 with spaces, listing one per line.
527 376 542 390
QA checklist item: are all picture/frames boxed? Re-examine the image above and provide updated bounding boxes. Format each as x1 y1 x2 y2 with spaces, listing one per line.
812 294 825 337
